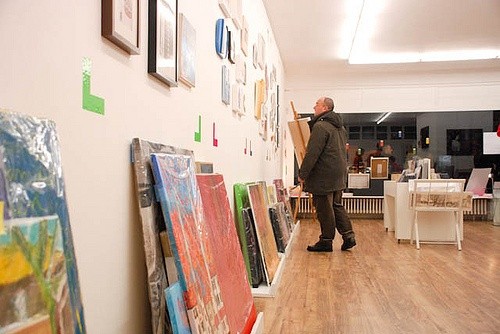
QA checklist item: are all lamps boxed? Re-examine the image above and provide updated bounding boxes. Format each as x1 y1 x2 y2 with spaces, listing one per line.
374 112 392 125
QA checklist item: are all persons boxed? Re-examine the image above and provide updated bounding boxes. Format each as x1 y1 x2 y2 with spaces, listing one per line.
354 148 365 167
389 156 402 172
297 97 357 252
367 147 382 166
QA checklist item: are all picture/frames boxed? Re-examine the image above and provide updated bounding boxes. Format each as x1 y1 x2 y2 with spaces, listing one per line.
147 0 179 87
347 173 370 190
179 12 196 88
370 157 389 180
217 0 268 120
102 0 141 55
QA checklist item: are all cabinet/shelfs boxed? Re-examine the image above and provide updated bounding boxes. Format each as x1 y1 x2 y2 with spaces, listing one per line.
408 178 466 251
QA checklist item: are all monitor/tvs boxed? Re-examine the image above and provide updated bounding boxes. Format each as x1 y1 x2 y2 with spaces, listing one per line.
446 128 483 156
420 126 429 149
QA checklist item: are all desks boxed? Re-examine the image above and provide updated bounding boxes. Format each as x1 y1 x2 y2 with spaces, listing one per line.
383 181 464 244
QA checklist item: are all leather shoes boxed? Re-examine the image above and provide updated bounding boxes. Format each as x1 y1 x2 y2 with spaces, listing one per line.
307 242 333 252
341 239 356 250
